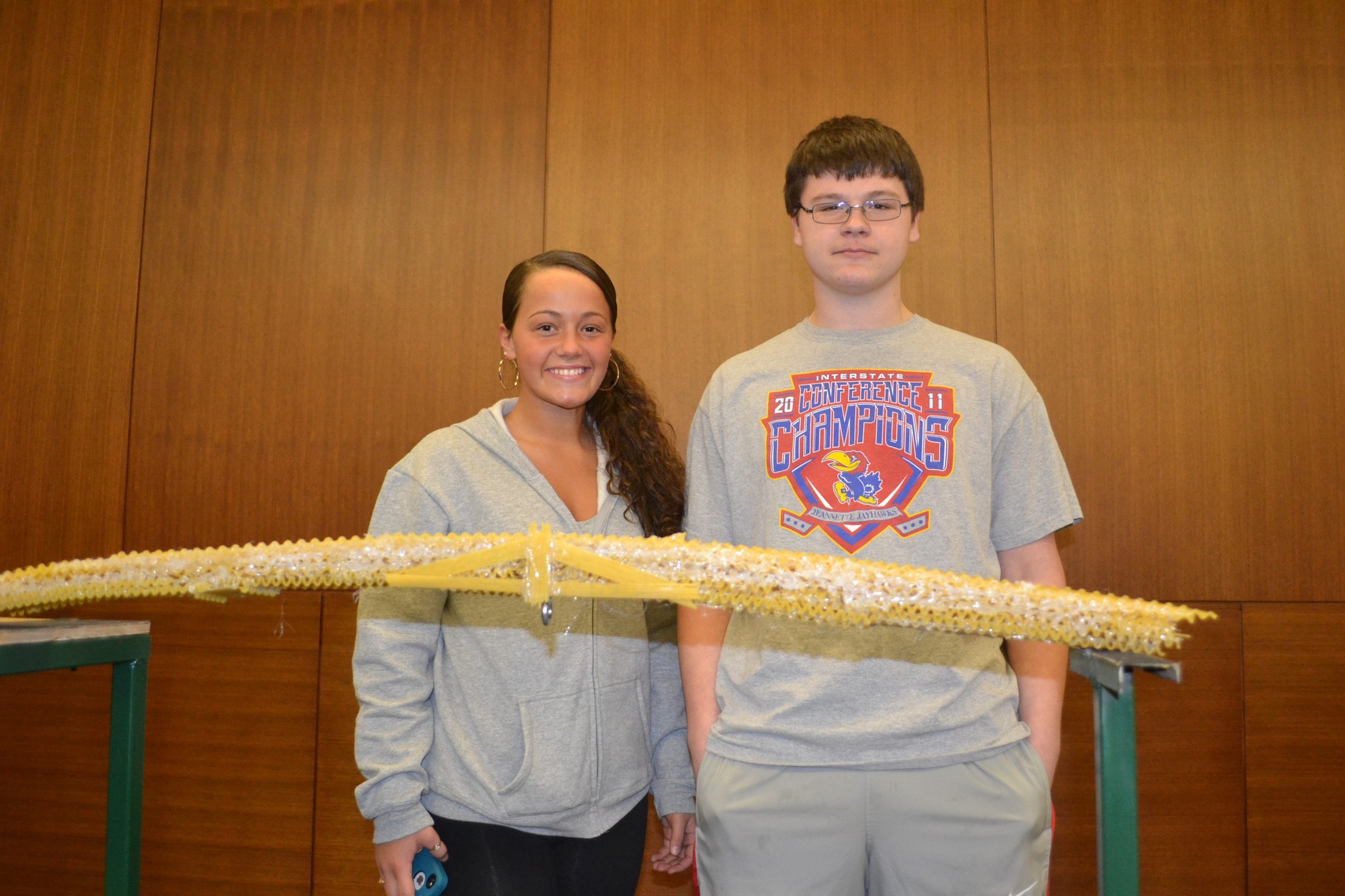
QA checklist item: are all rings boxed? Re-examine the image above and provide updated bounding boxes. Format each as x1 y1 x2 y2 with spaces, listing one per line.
429 840 442 852
379 878 385 884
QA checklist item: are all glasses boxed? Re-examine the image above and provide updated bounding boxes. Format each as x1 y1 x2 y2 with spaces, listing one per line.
800 199 912 224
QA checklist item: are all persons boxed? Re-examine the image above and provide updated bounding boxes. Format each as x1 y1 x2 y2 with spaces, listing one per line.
676 117 1084 896
352 252 697 896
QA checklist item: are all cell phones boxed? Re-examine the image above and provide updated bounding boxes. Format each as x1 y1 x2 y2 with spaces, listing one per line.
412 849 448 896
